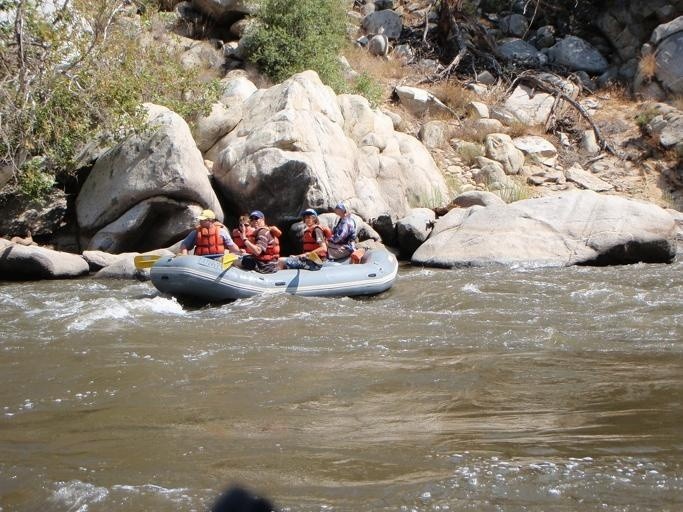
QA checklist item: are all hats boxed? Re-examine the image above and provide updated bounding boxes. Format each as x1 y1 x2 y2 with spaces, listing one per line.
194 208 216 221
248 210 264 220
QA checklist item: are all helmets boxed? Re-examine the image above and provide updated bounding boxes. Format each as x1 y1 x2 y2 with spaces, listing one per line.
301 207 318 219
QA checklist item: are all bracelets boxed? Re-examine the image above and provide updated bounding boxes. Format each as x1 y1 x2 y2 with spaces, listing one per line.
242 238 248 242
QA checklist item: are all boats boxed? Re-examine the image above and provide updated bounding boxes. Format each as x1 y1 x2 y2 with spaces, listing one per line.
150 250 399 304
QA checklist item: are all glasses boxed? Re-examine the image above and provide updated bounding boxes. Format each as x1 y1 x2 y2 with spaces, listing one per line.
240 224 250 227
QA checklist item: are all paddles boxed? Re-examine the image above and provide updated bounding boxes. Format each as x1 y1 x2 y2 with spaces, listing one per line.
222 253 252 269
292 253 323 264
135 254 224 269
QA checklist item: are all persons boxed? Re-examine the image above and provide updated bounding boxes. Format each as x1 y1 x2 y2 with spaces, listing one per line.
278 208 328 271
179 209 245 264
192 210 231 238
230 209 279 274
326 201 356 260
230 213 256 255
